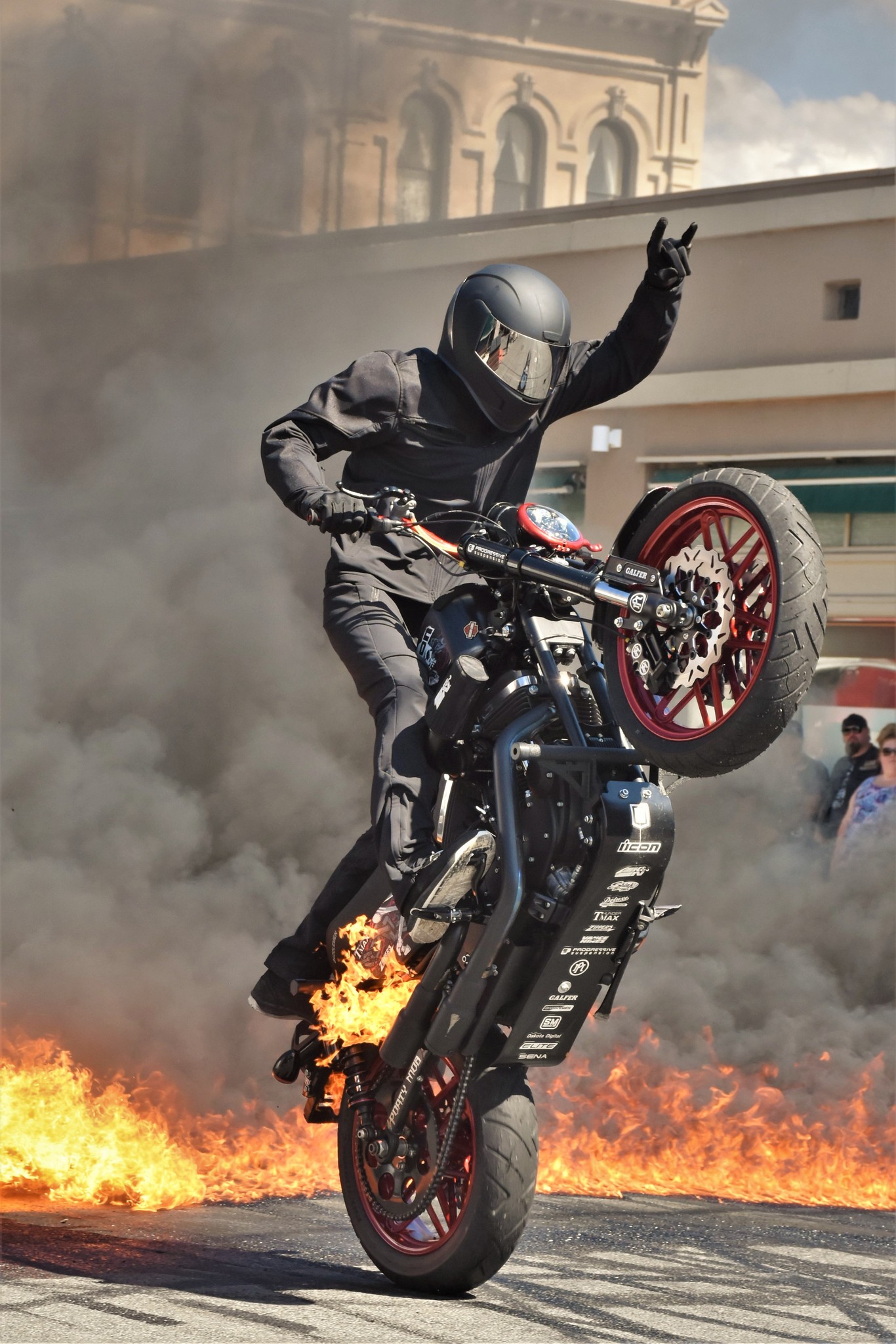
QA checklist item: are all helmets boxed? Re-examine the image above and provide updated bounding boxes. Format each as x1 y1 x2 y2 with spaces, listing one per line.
439 264 573 434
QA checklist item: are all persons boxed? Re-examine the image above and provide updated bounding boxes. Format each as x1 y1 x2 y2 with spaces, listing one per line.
249 215 702 1021
771 719 830 821
826 723 896 879
815 714 881 884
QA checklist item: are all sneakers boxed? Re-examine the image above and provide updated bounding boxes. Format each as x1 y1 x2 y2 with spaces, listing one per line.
402 827 496 945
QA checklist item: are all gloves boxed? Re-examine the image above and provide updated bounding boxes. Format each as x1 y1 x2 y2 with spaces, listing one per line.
644 215 698 291
310 491 364 534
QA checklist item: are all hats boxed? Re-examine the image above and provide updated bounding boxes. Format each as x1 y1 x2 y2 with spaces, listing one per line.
842 714 867 730
877 723 896 745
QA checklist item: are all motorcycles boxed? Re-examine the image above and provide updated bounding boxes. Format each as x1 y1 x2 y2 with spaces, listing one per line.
271 467 828 1308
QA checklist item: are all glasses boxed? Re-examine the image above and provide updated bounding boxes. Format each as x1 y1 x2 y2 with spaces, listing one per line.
883 747 896 756
842 726 862 734
